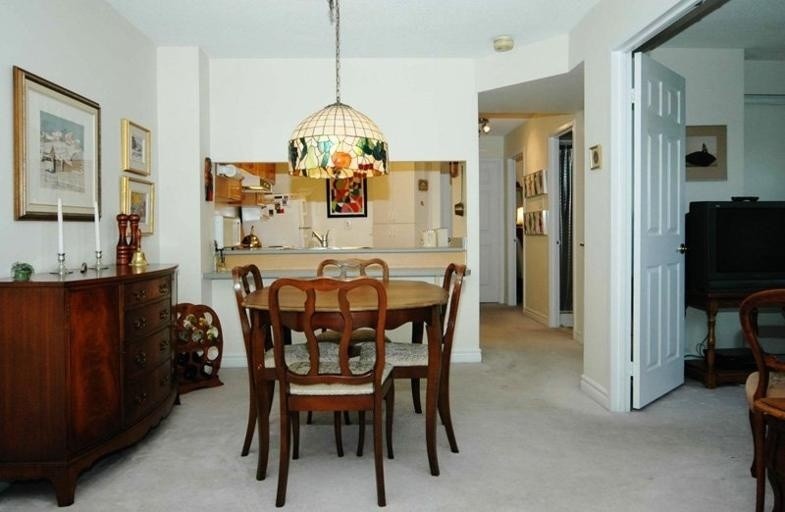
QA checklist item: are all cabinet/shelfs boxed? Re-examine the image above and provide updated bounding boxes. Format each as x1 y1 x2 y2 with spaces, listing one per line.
0 263 180 507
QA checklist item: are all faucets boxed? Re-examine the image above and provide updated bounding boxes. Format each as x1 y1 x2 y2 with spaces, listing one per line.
311 230 330 247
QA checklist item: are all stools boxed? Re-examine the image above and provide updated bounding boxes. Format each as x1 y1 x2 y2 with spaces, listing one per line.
754 396 784 457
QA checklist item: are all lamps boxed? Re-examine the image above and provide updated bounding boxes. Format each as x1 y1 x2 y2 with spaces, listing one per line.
517 206 525 227
288 1 387 180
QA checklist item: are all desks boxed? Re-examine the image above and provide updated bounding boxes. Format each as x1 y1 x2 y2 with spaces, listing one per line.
215 245 470 269
685 290 785 388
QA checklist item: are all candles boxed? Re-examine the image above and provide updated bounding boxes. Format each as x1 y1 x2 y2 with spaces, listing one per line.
92 200 101 254
56 198 65 255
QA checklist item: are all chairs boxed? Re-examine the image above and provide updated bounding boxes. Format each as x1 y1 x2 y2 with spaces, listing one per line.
357 262 463 458
268 277 395 508
739 289 785 458
317 257 389 343
231 264 345 458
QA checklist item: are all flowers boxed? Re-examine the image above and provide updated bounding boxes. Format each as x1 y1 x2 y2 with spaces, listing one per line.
175 313 218 345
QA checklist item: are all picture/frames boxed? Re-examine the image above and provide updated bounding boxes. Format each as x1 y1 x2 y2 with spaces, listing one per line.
120 118 153 177
522 210 547 236
326 175 367 218
520 169 549 198
685 124 729 183
590 143 603 171
12 65 103 223
122 172 157 236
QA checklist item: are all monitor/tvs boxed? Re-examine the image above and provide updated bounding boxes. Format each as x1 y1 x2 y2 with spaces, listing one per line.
684 200 785 292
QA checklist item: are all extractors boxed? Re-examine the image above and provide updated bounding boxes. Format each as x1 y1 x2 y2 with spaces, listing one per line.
239 167 272 194
